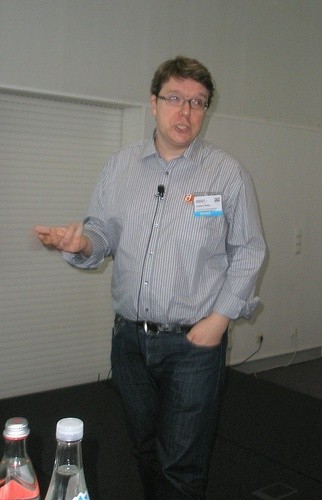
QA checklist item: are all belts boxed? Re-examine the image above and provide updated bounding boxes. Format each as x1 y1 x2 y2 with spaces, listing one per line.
115 316 195 333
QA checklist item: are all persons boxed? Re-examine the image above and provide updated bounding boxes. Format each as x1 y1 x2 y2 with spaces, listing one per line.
33 57 268 500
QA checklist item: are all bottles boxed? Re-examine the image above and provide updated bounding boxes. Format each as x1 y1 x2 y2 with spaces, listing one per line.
0 417 41 500
44 417 90 500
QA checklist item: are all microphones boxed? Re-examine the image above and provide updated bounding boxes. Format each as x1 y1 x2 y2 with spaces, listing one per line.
157 185 165 197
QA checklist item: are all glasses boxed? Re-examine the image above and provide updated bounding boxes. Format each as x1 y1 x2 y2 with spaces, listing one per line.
156 95 209 110
